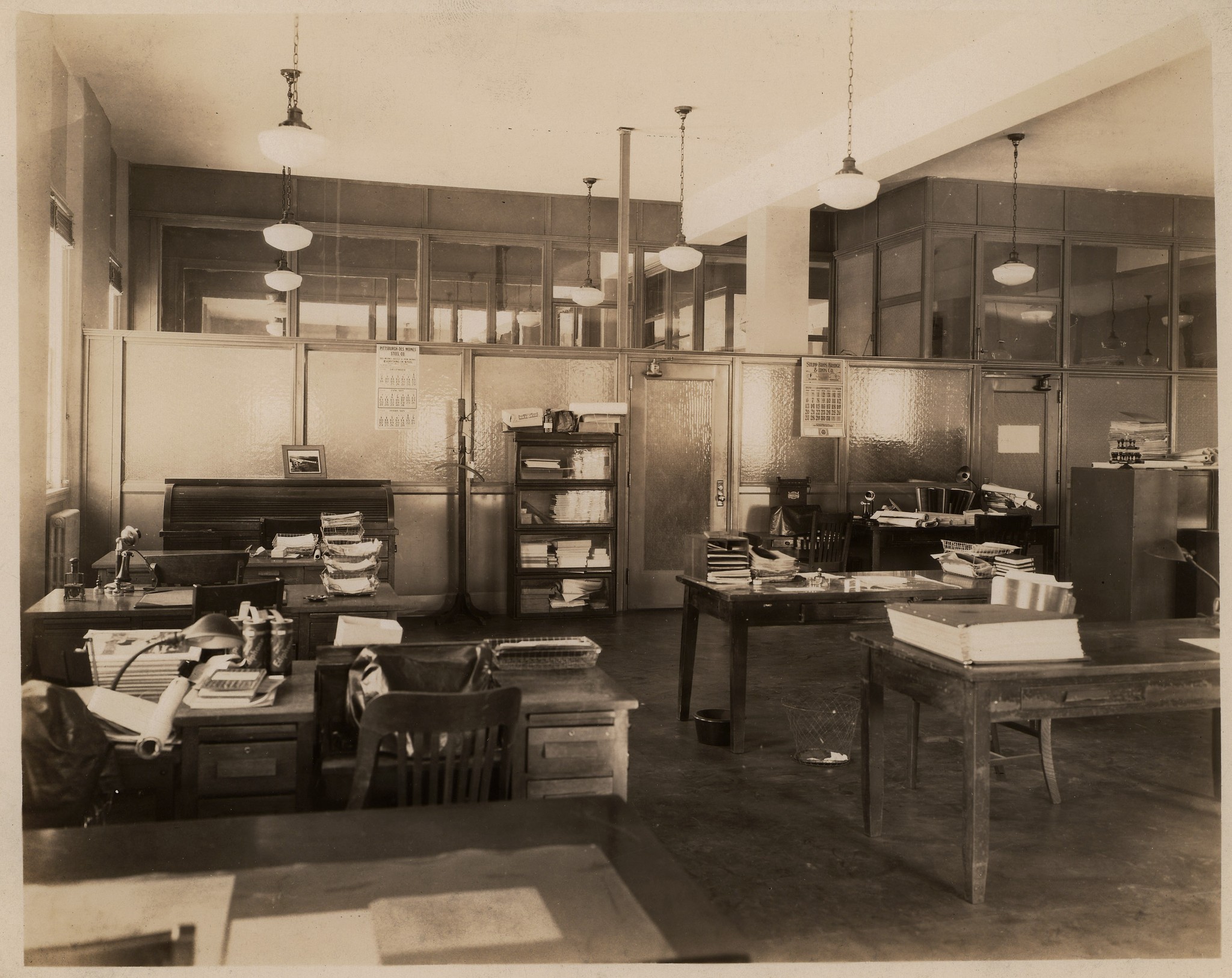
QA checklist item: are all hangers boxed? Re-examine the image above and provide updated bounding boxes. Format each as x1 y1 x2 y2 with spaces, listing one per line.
433 416 487 451
435 452 485 482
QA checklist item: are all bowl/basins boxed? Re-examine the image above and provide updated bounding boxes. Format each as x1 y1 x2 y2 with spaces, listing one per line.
692 708 747 746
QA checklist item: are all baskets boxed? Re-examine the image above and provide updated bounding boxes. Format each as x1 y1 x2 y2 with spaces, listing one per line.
484 634 603 671
272 533 319 555
320 512 382 596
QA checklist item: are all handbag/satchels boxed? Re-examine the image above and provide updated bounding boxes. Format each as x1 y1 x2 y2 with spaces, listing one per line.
345 639 496 760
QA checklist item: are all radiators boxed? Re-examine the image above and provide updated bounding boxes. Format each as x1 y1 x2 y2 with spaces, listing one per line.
47 508 80 593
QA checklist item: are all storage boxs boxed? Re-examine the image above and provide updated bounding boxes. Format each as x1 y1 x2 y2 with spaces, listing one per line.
502 407 543 428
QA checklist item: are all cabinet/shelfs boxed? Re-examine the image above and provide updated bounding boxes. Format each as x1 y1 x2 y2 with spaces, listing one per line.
682 534 750 585
506 432 618 619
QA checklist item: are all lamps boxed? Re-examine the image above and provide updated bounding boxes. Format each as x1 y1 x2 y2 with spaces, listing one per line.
1137 295 1159 366
819 11 881 211
1143 537 1219 615
1161 305 1194 329
266 292 285 318
993 133 1035 287
263 69 312 252
1021 244 1053 325
258 14 330 166
266 318 283 336
265 165 302 292
572 177 605 307
1100 280 1126 350
658 105 703 272
516 247 540 327
956 466 981 509
111 614 245 690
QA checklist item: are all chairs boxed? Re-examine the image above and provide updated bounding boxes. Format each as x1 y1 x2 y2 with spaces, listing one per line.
909 575 1074 806
259 516 323 550
795 511 854 573
191 579 286 627
348 686 522 810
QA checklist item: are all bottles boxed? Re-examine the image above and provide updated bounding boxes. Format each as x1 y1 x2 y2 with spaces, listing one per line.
543 409 553 433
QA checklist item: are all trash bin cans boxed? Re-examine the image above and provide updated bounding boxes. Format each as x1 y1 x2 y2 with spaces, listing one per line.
782 690 861 766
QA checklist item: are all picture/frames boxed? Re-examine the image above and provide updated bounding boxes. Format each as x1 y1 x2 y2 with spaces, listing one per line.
282 444 328 479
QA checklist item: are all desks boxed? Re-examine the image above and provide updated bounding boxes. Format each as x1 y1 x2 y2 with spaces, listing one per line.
172 659 637 813
24 793 751 970
848 616 1221 904
847 519 1059 574
743 531 844 551
674 569 993 755
23 582 417 686
91 550 329 585
